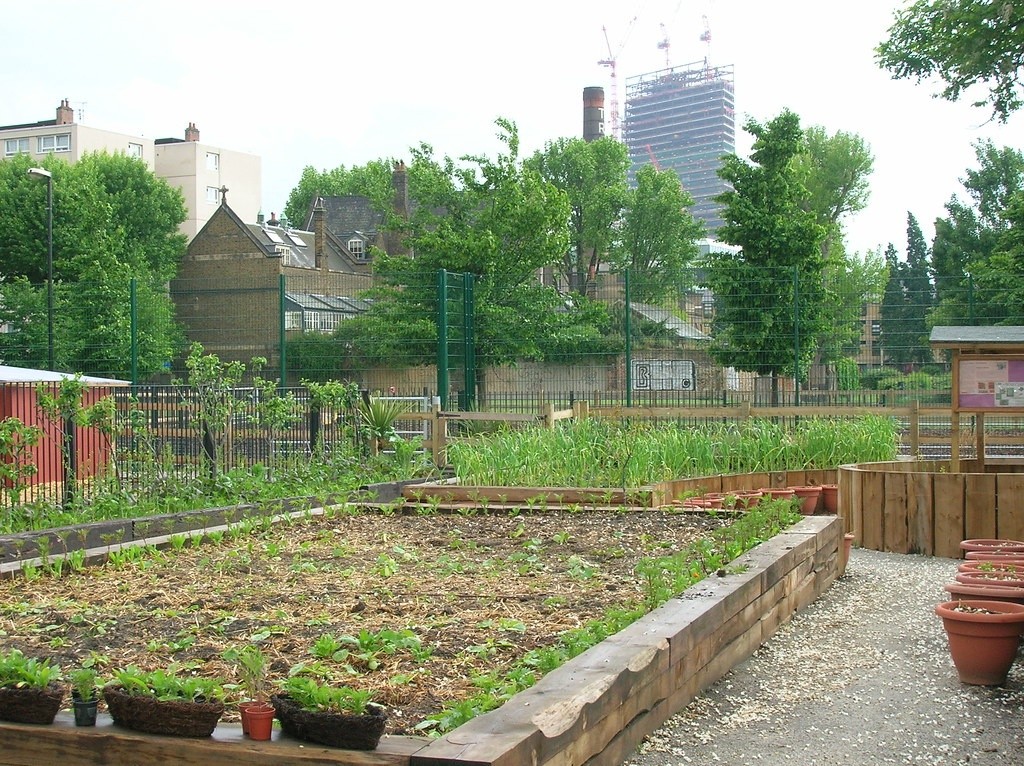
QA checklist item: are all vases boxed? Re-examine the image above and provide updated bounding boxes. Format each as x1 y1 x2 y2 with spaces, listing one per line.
945 583 1024 605
965 552 1024 560
843 533 855 570
663 483 838 516
956 574 1024 587
960 539 1024 553
935 599 1024 685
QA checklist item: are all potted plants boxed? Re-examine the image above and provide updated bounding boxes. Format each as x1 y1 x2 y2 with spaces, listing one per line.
102 662 226 737
0 649 66 725
67 669 100 727
959 562 1024 577
270 675 387 750
226 647 276 741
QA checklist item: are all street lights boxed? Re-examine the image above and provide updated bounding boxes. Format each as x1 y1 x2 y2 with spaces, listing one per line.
27 167 55 371
